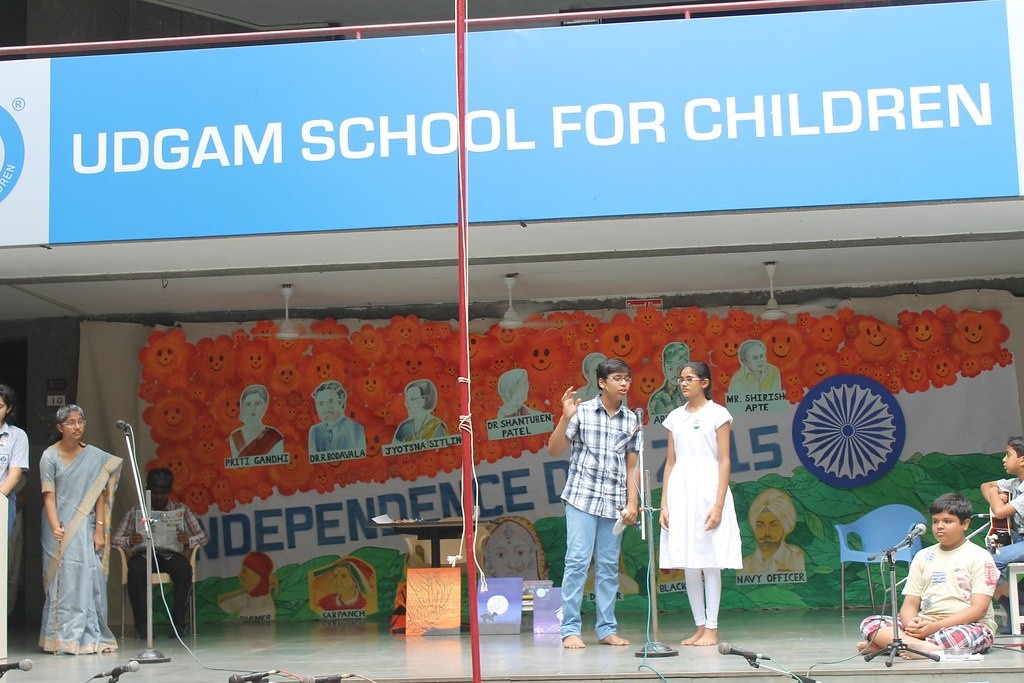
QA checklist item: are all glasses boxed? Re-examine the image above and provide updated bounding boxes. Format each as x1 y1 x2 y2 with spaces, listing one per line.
61 419 87 427
677 377 704 384
604 376 632 384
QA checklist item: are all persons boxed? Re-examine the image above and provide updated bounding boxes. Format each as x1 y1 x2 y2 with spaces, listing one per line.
659 362 743 646
856 493 1001 660
0 384 29 540
113 468 209 637
39 405 118 655
549 358 640 648
981 436 1024 634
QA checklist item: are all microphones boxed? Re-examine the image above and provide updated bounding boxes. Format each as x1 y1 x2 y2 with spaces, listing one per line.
303 674 356 683
229 670 281 683
718 642 771 659
905 524 927 541
973 514 990 519
94 661 140 678
116 420 131 430
0 659 33 673
635 408 644 427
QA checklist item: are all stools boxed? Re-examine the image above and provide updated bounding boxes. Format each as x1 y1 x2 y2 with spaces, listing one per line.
1007 560 1024 635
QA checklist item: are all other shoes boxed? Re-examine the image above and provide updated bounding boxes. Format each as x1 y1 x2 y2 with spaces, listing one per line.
172 626 186 639
138 629 155 639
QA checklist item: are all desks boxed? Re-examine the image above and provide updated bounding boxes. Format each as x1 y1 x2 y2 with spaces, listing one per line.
358 519 504 636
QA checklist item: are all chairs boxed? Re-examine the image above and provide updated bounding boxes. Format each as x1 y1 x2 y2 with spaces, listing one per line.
106 531 209 642
834 505 929 620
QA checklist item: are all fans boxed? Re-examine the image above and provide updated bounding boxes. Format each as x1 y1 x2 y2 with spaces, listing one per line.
230 283 348 340
716 262 840 319
467 274 579 330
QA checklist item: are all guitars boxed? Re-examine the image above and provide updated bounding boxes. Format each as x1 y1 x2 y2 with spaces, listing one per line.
985 490 1012 555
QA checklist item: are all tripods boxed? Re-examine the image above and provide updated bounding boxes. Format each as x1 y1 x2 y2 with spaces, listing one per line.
864 541 941 666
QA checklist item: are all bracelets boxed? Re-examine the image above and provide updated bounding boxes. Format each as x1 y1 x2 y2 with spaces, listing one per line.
96 521 103 525
